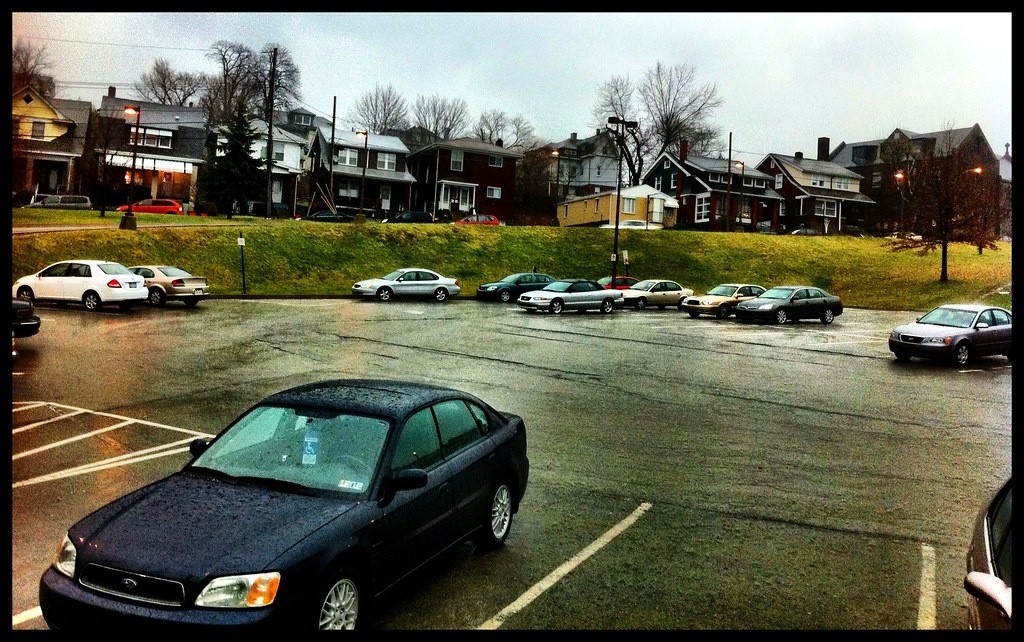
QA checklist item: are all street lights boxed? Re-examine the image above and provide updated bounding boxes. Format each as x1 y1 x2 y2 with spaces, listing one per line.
895 169 905 230
124 106 141 214
552 150 561 204
735 161 745 224
356 131 367 212
608 116 638 288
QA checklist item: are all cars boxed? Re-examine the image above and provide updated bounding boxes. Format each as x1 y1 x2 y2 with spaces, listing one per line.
962 477 1015 631
517 275 694 316
11 259 149 310
351 268 460 302
295 209 352 222
11 297 41 337
681 283 843 325
889 304 1012 367
116 198 182 214
476 272 556 302
37 378 531 631
381 209 500 225
128 265 209 308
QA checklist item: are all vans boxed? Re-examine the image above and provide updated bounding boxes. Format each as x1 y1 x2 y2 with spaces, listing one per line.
21 195 93 210
